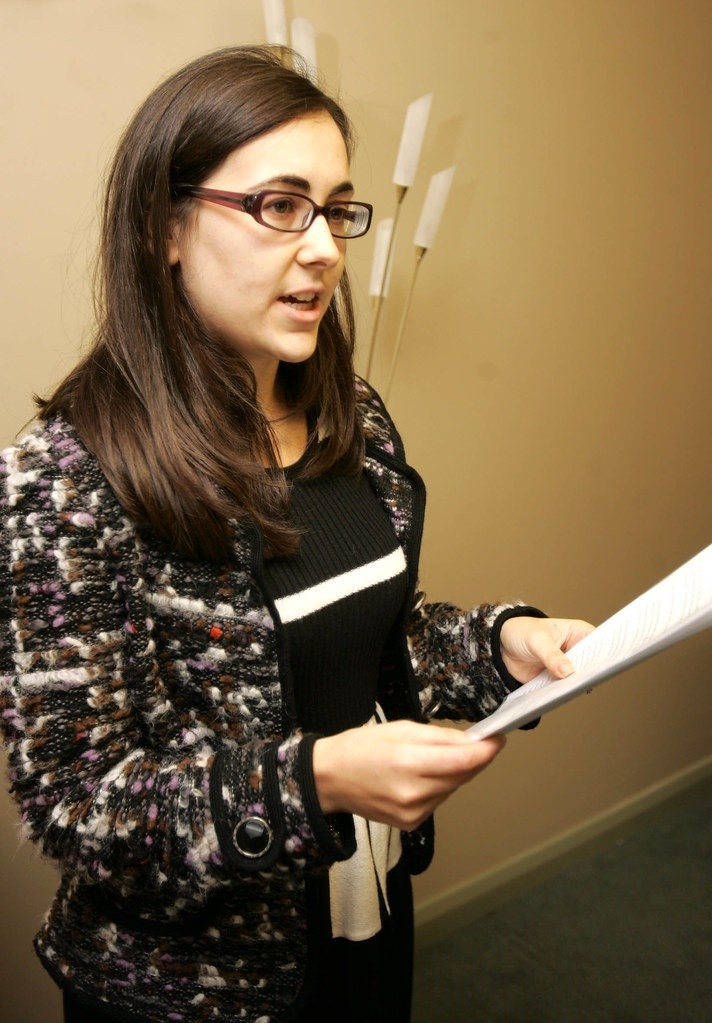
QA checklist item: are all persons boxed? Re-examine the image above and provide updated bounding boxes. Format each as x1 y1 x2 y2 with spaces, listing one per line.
0 48 598 1023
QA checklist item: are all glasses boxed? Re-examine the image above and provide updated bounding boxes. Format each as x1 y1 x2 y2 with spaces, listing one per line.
173 184 374 238
